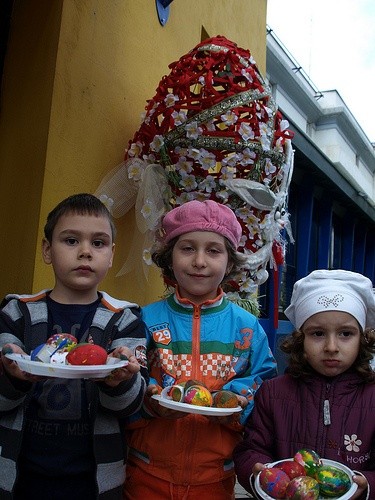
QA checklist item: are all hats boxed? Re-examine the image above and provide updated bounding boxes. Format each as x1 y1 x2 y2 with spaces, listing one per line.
162 201 242 252
284 269 375 334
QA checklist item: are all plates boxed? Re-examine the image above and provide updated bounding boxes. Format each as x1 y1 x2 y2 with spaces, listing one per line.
5 353 129 379
151 395 242 417
254 458 358 500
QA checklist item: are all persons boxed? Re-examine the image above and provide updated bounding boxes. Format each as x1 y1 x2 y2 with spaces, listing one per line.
121 199 279 500
0 192 148 500
235 270 375 500
